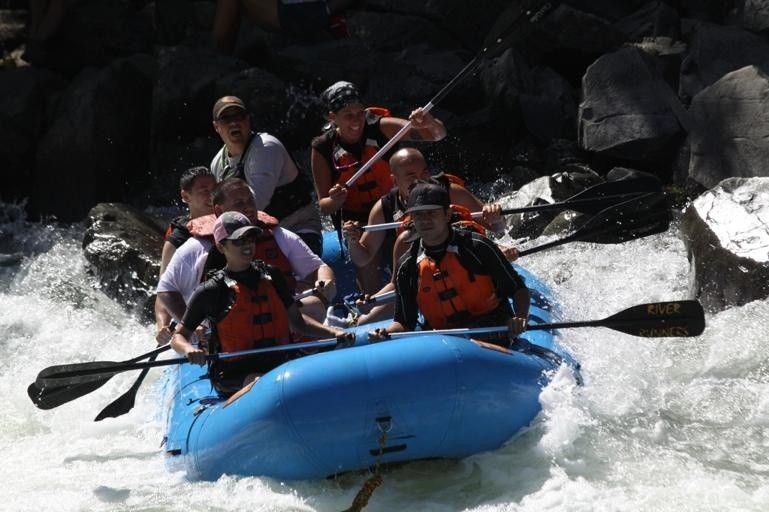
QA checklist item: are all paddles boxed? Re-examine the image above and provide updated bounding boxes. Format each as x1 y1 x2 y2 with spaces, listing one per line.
94 318 175 422
370 299 704 337
343 0 560 189
26 325 175 409
365 184 675 303
353 179 666 233
35 332 354 389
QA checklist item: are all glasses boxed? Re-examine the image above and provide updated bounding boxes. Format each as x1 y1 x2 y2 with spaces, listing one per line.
218 112 245 124
232 234 257 246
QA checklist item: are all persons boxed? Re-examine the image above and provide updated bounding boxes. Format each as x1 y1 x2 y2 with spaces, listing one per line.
342 147 509 273
170 211 357 401
155 177 339 356
311 79 448 302
208 95 323 259
156 166 220 349
355 177 519 326
368 183 531 349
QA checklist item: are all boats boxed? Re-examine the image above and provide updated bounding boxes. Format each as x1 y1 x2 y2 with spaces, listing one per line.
162 258 584 486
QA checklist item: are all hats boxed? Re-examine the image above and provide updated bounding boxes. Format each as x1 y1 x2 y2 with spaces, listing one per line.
213 96 247 120
404 183 450 214
325 82 362 113
212 211 263 243
